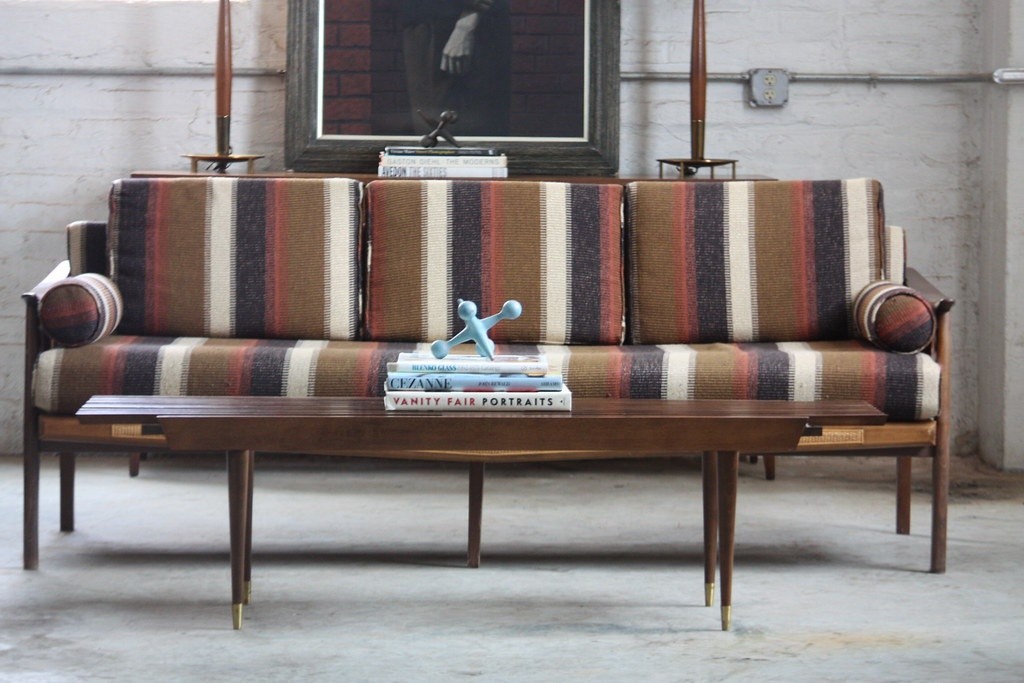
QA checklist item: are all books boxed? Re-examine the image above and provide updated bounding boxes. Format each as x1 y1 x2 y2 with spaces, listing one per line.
377 146 509 179
383 353 573 412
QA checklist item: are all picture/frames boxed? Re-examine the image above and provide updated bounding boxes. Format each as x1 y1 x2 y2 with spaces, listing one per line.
286 0 621 174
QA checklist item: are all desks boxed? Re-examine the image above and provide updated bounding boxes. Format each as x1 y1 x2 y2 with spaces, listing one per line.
76 396 889 634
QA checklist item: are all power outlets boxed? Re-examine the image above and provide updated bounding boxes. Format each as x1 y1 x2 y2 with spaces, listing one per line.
746 67 789 106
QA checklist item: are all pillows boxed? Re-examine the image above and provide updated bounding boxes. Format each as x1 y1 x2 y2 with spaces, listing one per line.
109 177 360 342
627 178 888 344
852 280 937 354
363 178 624 344
39 272 122 347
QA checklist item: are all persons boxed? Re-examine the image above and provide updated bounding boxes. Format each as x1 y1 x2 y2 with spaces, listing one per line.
397 0 512 131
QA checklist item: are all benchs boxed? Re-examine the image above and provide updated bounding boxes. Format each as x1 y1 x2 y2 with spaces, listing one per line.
22 221 958 575
